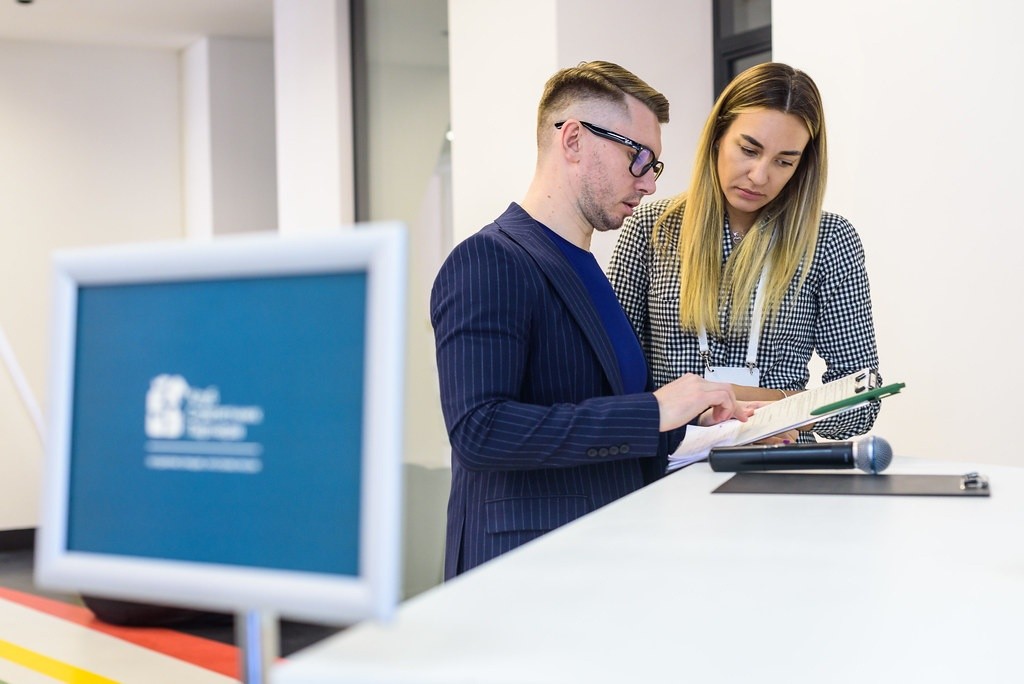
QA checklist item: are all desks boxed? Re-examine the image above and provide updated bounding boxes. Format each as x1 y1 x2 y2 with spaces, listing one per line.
263 446 1022 684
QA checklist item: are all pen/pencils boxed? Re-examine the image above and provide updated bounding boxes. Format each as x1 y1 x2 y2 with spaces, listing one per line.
808 382 906 416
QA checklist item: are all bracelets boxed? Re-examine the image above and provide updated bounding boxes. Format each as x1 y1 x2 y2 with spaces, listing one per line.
777 389 787 398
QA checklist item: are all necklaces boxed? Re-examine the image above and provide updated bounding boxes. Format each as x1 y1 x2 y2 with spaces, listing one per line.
731 230 745 245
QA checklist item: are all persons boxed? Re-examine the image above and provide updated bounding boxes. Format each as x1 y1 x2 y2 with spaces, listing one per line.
430 59 779 584
605 62 884 443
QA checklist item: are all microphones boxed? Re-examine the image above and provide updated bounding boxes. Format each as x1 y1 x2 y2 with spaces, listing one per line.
708 435 891 474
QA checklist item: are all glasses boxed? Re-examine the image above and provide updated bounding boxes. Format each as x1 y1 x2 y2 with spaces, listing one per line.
555 119 664 182
